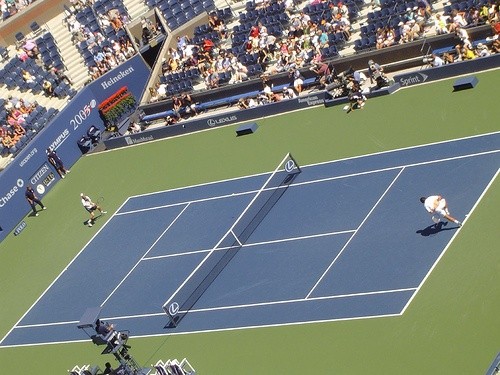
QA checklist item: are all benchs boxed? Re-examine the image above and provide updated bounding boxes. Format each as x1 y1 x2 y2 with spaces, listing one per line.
271 75 318 93
431 37 492 58
168 101 199 121
200 95 231 112
141 109 171 126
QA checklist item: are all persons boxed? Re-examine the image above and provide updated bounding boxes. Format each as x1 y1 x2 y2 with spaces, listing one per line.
0 0 500 147
419 194 462 229
80 193 108 228
48 148 70 179
94 319 114 337
25 185 47 217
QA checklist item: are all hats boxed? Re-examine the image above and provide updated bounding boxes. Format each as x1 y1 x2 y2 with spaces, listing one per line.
336 14 341 18
321 20 326 24
368 61 374 66
410 19 415 23
413 6 418 10
406 8 411 11
398 22 404 26
291 15 295 19
477 43 483 49
81 193 84 196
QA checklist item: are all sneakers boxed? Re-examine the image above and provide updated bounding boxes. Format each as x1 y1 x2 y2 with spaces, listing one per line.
36 213 38 216
43 207 46 209
87 222 93 226
458 222 462 226
100 211 107 213
66 170 70 173
62 176 65 178
448 212 450 215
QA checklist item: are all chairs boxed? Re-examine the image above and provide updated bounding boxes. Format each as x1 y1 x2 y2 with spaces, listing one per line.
210 8 232 21
145 0 214 28
151 359 171 375
29 21 41 32
239 55 264 74
81 365 103 375
14 31 26 42
258 2 288 34
90 330 130 355
87 125 104 142
443 0 481 13
65 0 131 68
35 32 62 69
193 25 219 44
303 2 329 21
226 10 258 54
0 98 59 155
218 72 231 82
332 0 361 17
67 364 86 375
244 2 256 11
320 32 347 57
169 358 195 375
0 47 74 97
354 0 414 50
159 68 199 93
78 135 97 152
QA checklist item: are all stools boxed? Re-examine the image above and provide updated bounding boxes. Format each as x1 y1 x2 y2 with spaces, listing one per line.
453 76 479 90
235 122 257 134
231 90 264 105
